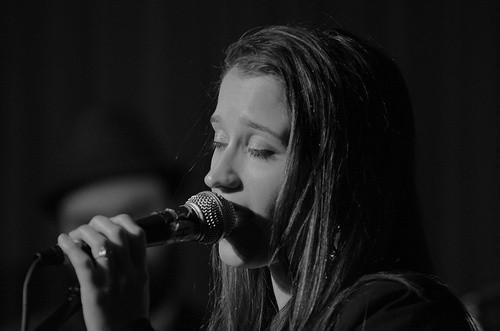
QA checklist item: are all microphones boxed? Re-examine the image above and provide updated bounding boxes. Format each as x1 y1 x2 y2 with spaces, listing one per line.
35 188 237 272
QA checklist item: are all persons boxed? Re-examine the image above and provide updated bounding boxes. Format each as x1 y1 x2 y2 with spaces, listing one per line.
57 21 481 331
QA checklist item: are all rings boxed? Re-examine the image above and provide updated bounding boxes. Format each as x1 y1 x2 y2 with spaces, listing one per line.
93 246 115 260
